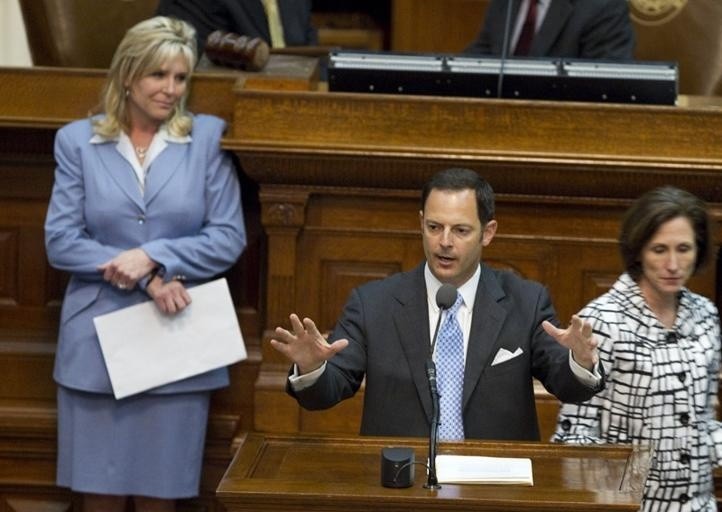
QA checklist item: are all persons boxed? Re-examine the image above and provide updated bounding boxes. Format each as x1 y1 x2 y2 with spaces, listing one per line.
156 1 316 71
42 15 252 512
268 166 606 447
548 186 722 511
456 1 637 63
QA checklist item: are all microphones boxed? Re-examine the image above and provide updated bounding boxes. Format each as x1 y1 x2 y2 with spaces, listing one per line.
415 284 458 491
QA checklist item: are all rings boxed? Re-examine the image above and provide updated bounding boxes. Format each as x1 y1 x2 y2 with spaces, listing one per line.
117 281 127 289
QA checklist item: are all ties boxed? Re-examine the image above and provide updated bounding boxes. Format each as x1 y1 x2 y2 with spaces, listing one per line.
432 294 466 444
261 0 285 48
511 0 544 57
